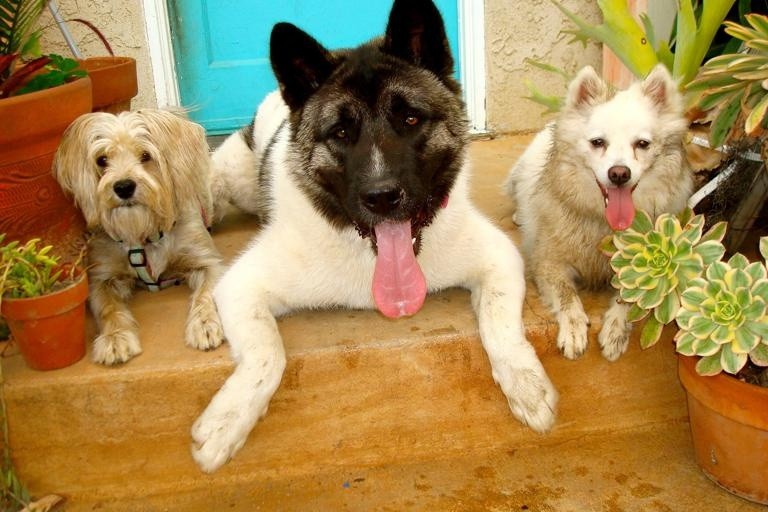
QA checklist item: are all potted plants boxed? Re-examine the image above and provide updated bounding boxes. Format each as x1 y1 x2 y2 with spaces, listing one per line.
0 0 115 247
601 211 767 509
0 231 88 372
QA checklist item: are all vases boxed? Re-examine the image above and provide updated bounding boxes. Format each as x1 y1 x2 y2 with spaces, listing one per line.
53 56 138 115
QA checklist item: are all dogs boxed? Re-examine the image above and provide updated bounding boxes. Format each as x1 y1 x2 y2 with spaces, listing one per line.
500 63 696 363
48 104 230 370
186 0 562 478
